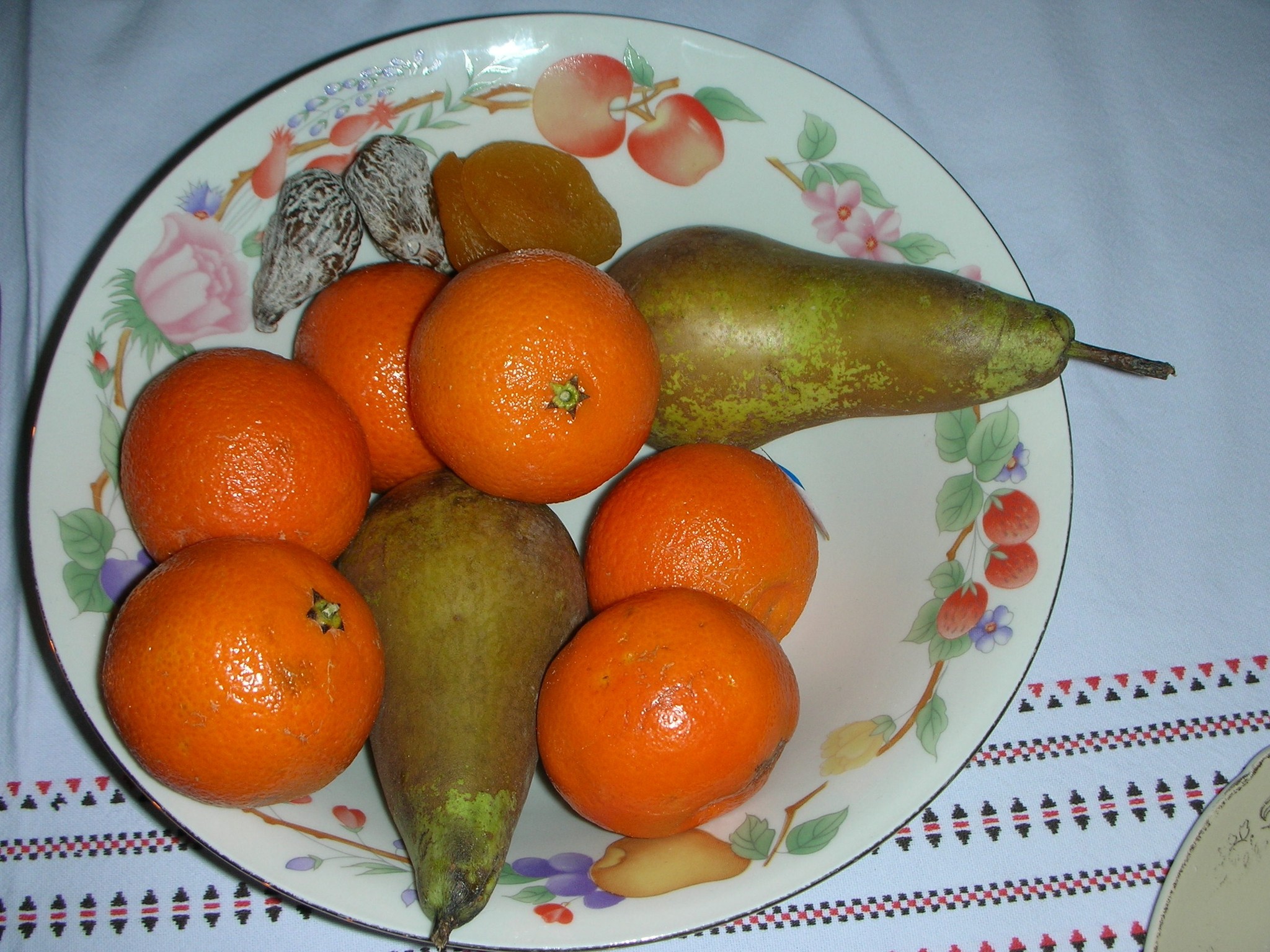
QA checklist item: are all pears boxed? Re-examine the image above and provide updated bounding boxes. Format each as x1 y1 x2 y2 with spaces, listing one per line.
338 468 588 949
603 224 1176 453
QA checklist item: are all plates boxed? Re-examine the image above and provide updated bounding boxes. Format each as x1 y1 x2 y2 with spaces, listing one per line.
1144 746 1270 952
27 11 1074 952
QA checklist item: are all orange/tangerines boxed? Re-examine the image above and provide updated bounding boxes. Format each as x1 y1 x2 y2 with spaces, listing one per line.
292 263 450 494
587 441 819 647
120 348 370 565
102 538 384 807
407 248 660 505
537 586 798 839
